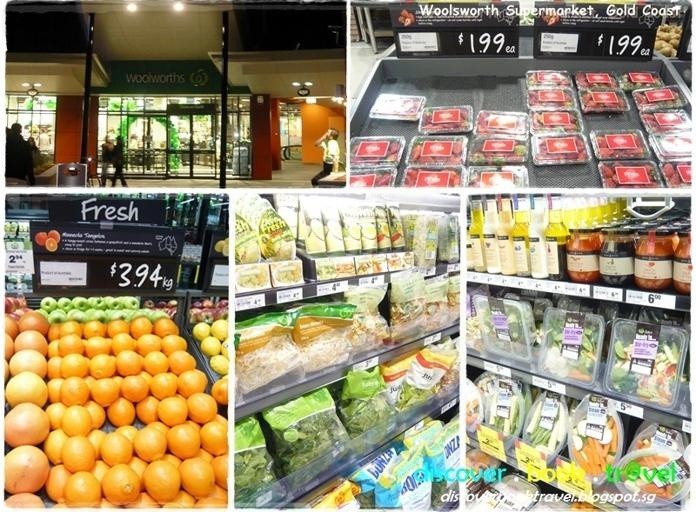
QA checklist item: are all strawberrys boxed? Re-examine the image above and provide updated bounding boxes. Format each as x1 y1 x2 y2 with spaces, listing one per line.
350 71 692 188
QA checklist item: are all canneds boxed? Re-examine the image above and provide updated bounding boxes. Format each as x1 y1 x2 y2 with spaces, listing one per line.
566 215 691 294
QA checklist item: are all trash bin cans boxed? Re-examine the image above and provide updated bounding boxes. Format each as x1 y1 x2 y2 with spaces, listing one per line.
57 164 87 188
231 147 248 176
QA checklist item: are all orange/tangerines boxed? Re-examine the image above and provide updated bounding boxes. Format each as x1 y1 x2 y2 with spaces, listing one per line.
4 309 227 509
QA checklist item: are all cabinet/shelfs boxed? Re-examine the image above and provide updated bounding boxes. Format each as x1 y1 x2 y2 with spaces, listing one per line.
462 267 692 509
235 258 461 511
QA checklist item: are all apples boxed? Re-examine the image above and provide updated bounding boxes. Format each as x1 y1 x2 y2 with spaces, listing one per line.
5 295 228 325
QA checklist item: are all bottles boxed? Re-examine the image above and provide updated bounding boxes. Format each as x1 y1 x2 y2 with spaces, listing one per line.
466 198 632 280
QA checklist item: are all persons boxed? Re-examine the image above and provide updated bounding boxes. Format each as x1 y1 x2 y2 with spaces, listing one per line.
6 123 36 186
101 136 113 186
311 128 340 185
110 136 127 187
26 128 51 168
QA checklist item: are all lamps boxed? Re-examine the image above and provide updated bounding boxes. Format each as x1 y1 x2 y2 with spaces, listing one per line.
296 83 311 96
25 82 39 97
